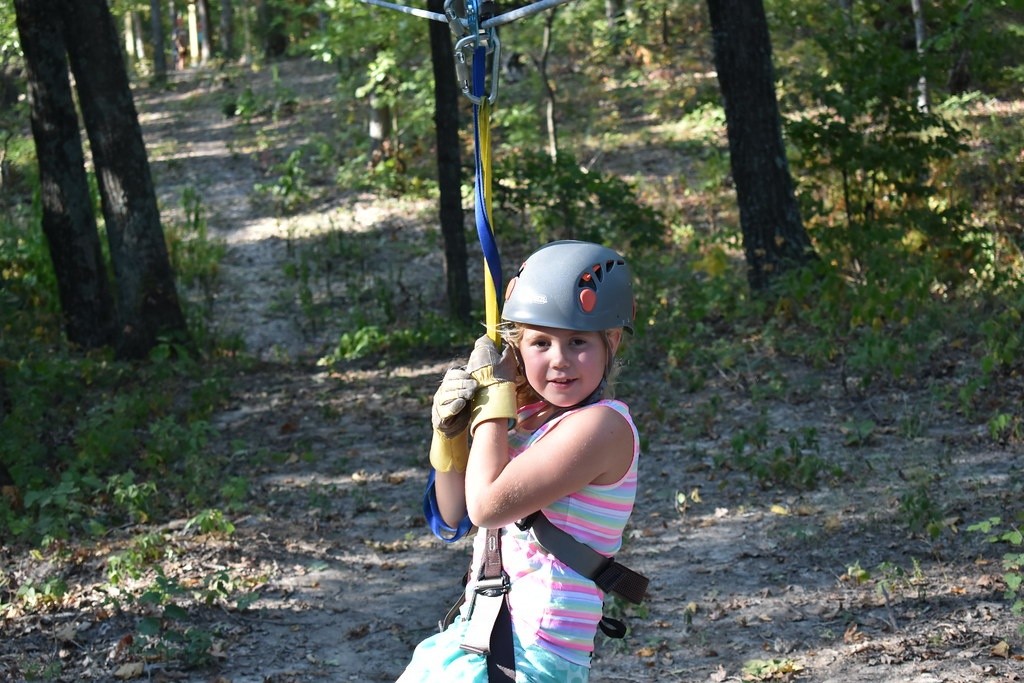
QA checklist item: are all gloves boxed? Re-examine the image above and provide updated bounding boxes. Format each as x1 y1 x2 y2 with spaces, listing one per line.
429 365 479 472
465 334 522 436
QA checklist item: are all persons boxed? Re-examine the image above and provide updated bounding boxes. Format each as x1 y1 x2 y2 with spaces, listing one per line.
393 238 641 683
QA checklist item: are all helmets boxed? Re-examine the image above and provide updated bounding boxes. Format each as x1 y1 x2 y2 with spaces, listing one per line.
501 240 635 335
